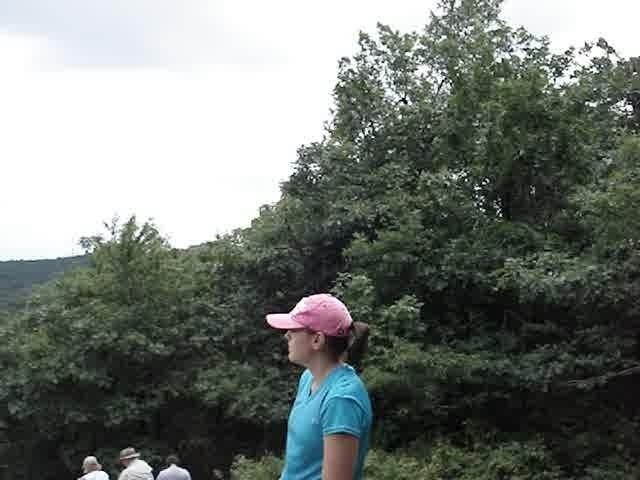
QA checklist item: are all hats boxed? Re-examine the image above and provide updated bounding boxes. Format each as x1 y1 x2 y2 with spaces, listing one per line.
265 292 353 337
120 447 140 460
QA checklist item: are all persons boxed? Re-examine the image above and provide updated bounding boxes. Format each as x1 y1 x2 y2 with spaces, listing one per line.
77 455 109 480
265 292 373 480
156 456 192 480
117 447 154 480
